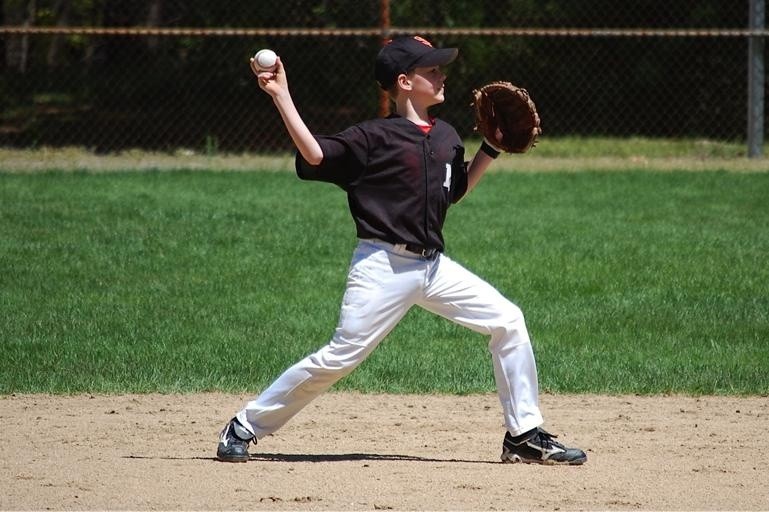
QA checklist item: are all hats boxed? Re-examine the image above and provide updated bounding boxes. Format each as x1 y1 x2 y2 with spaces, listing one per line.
373 36 459 88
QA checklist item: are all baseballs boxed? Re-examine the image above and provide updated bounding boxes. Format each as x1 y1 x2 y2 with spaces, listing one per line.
253 49 278 73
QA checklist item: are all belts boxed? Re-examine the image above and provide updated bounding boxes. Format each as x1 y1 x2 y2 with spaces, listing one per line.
405 243 440 262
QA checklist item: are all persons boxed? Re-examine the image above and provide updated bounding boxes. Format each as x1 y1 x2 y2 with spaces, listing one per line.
218 37 588 464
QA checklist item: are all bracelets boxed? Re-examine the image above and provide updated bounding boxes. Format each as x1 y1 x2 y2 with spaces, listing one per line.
481 141 500 159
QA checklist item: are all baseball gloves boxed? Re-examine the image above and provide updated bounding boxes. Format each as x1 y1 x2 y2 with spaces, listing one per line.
470 80 543 154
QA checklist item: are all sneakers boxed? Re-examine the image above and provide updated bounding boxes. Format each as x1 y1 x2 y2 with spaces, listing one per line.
217 418 257 462
500 427 588 466
